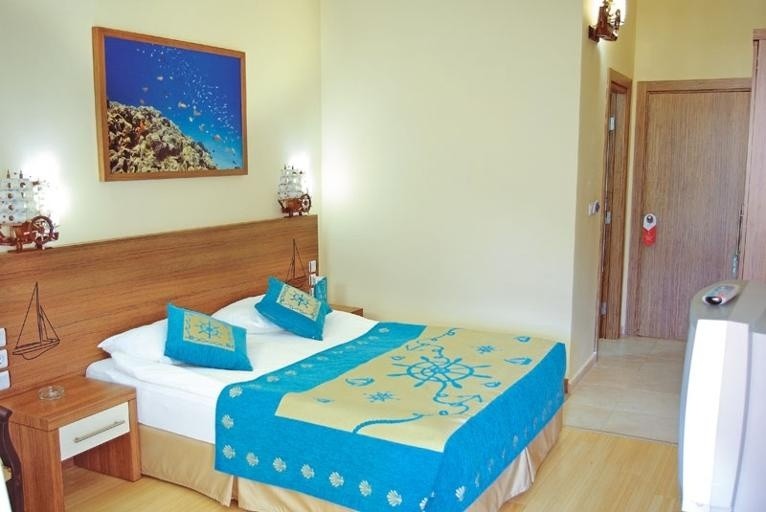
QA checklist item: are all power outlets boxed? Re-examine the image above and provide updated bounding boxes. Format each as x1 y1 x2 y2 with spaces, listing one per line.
0 326 11 391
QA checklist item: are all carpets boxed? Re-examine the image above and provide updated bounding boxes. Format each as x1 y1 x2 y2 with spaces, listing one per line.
567 337 686 443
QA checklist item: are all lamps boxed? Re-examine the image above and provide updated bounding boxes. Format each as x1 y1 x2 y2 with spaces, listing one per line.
588 0 626 43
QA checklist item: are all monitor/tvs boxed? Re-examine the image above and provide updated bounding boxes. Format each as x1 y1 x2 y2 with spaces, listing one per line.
680 279 766 512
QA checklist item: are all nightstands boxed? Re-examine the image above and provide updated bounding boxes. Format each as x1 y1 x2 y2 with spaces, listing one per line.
328 303 363 317
0 376 142 512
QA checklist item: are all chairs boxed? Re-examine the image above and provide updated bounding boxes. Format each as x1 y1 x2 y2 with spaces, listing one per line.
0 405 25 512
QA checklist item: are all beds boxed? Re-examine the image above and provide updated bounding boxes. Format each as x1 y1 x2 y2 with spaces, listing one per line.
86 310 566 512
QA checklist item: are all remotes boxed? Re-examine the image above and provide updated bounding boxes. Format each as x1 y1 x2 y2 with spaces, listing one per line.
703 283 740 306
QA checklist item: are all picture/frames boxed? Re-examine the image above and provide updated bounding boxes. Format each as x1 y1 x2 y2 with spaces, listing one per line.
92 27 248 183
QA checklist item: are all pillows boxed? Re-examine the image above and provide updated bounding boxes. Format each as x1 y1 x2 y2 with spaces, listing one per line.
96 275 333 371
